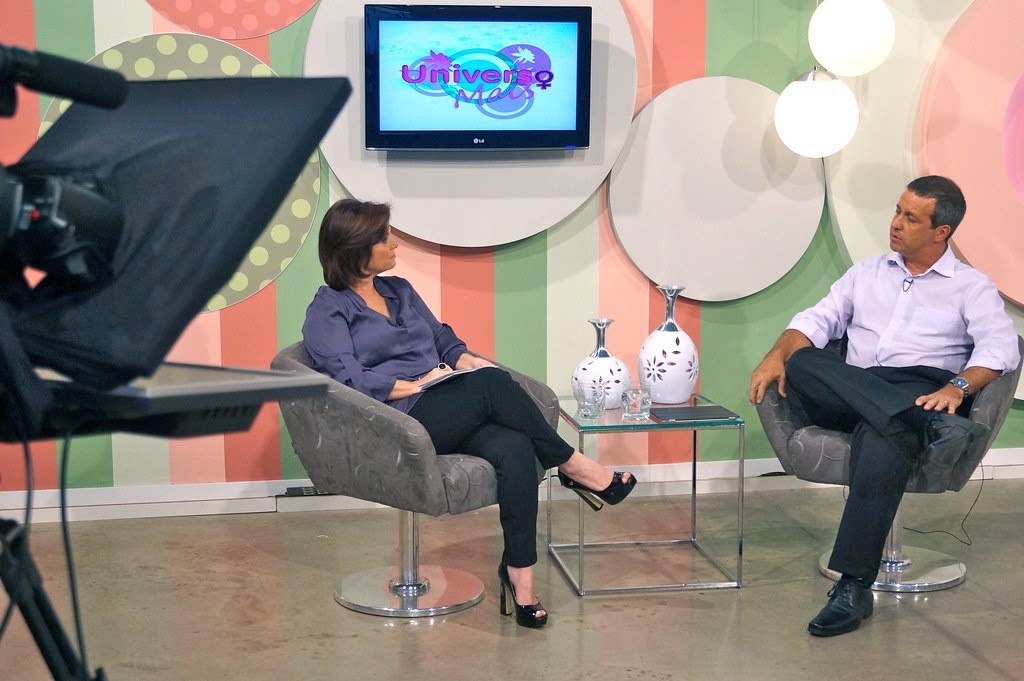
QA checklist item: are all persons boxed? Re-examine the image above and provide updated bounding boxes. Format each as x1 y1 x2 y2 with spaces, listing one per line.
748 172 1022 639
301 199 636 627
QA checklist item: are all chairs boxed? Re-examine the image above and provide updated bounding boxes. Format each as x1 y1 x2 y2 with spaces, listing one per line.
268 340 560 618
754 331 1024 591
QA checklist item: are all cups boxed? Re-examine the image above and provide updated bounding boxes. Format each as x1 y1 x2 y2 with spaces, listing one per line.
622 381 653 422
578 381 606 419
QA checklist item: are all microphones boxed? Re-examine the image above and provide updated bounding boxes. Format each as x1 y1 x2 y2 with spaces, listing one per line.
0 45 128 108
395 316 405 328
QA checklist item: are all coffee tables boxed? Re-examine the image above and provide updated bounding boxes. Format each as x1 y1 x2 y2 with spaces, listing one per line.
547 391 747 596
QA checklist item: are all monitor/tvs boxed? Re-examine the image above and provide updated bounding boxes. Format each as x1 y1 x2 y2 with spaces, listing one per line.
364 4 592 154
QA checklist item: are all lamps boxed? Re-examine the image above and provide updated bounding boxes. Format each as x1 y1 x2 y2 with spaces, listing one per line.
771 62 860 158
808 1 896 80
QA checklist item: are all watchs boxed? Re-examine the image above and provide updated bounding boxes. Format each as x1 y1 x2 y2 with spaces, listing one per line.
947 376 970 399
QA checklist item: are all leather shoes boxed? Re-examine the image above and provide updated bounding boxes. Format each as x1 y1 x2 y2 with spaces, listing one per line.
926 414 989 468
808 579 878 635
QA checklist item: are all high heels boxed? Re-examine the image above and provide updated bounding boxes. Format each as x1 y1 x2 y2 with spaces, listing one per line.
558 469 636 512
497 559 549 628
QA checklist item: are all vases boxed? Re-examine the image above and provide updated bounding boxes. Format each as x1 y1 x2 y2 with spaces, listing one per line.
637 284 699 405
570 317 632 412
